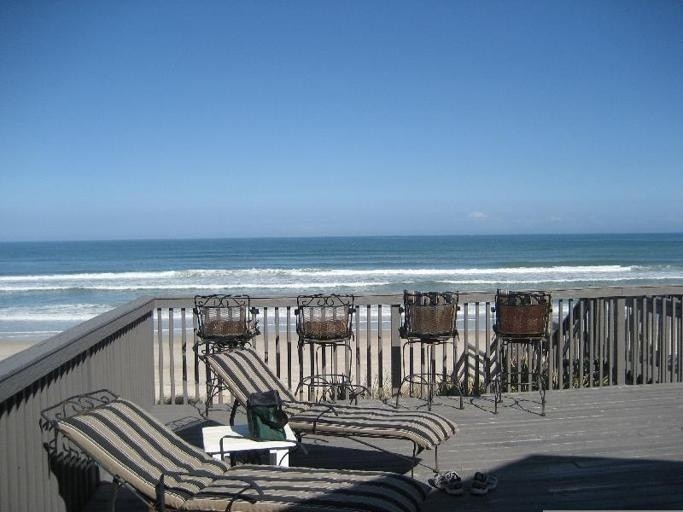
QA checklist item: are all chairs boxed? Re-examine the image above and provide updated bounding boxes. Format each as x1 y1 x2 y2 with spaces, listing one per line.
293 292 366 407
193 293 261 418
493 289 553 416
201 342 460 481
396 290 465 411
40 389 432 512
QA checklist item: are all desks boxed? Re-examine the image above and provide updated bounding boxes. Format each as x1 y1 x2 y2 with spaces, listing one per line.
202 422 298 466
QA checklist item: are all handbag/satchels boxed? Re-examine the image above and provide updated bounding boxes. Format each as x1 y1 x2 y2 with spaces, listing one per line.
245 389 289 441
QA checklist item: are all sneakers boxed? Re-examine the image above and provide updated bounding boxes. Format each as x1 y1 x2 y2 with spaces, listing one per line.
471 471 498 495
432 470 463 495
